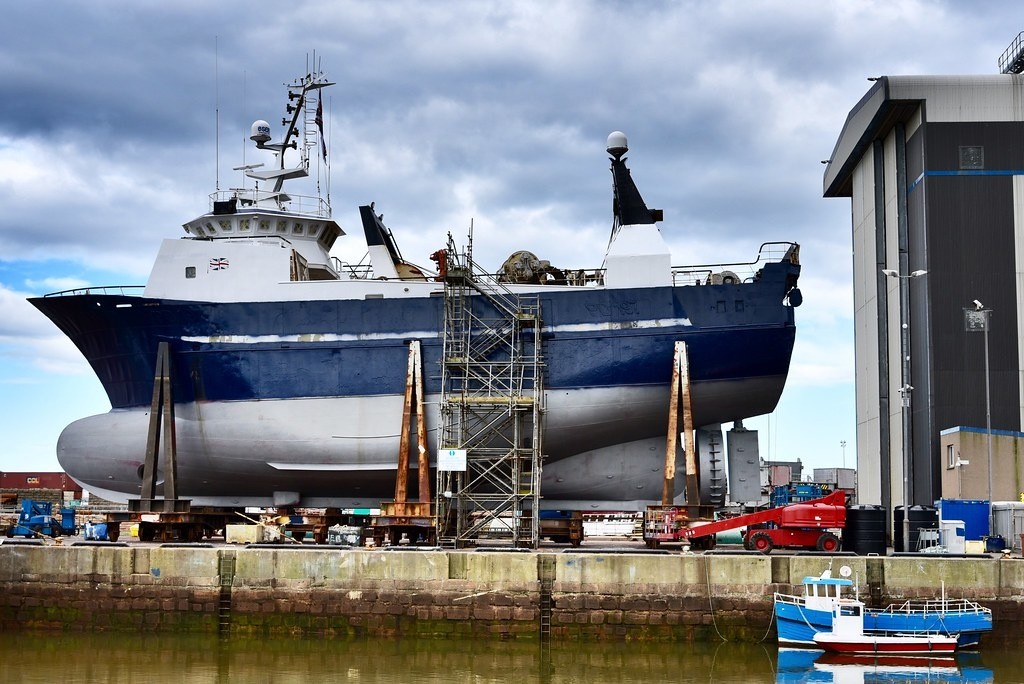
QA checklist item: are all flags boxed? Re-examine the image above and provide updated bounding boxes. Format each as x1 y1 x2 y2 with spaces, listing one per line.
315 88 327 165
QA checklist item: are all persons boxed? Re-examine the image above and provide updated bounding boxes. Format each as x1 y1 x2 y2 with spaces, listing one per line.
567 269 585 286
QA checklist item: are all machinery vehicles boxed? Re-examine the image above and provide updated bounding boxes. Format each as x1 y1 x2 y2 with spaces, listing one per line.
672 488 846 554
6 499 80 539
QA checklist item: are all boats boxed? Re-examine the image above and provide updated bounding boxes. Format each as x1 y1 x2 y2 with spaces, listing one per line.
810 597 962 654
774 647 996 684
24 33 804 509
771 556 993 649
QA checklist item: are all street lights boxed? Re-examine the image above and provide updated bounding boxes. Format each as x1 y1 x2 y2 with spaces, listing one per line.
840 440 848 469
974 299 995 536
882 267 930 553
